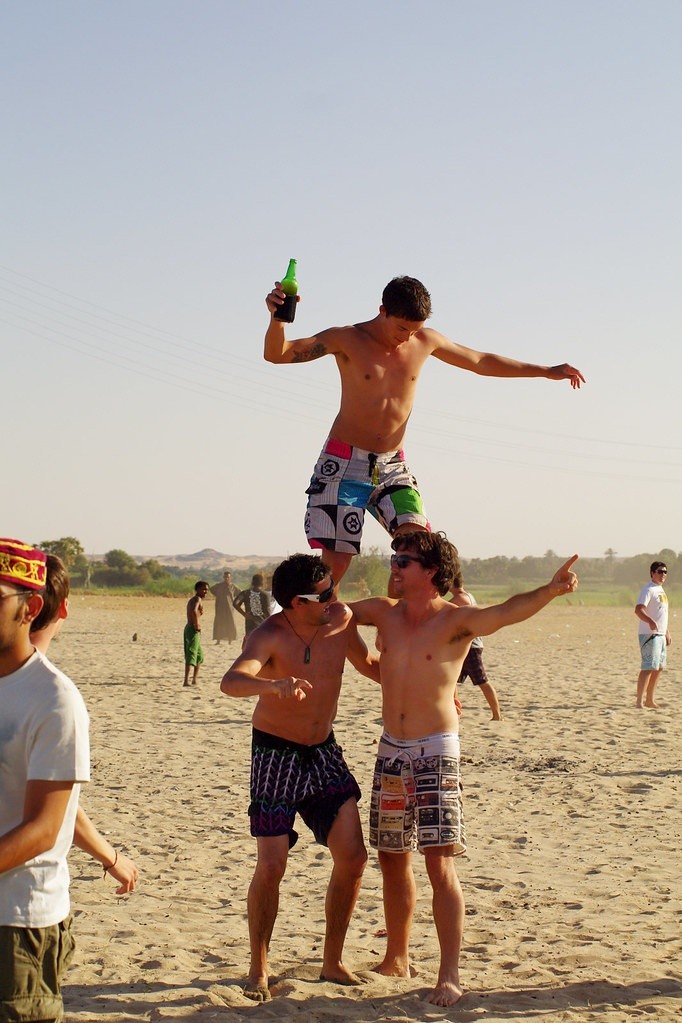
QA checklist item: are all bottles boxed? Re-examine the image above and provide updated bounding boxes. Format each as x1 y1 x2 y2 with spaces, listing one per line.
274 258 298 323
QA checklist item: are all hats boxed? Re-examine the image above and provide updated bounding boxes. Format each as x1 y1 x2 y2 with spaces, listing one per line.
0 537 47 591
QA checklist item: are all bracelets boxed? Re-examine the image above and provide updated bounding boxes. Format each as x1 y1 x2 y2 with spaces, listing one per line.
103 849 118 880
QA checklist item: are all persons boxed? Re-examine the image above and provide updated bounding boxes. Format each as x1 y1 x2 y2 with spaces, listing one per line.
0 538 139 1023
220 554 462 999
264 275 586 588
446 572 503 721
635 561 672 708
338 530 577 1007
183 581 209 686
209 571 243 645
233 575 270 636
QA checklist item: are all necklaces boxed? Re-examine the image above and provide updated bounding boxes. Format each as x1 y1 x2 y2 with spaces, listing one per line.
283 610 319 664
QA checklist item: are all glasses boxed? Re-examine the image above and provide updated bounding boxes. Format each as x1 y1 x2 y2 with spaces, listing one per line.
390 554 423 569
654 570 667 574
297 579 335 604
